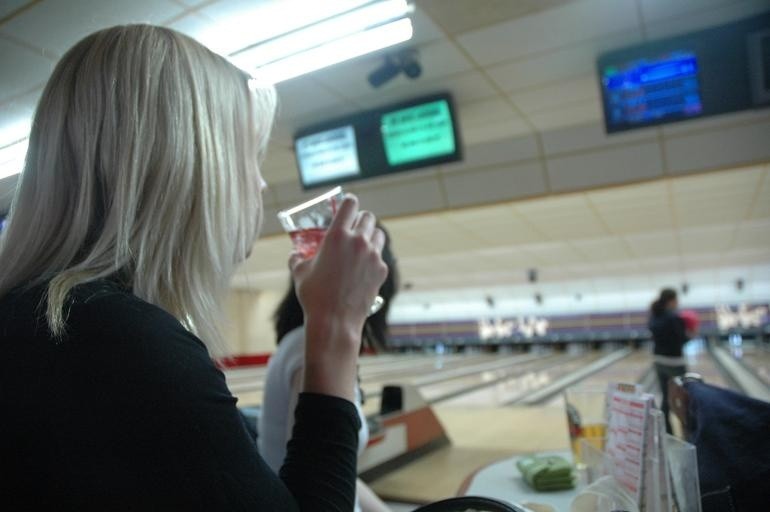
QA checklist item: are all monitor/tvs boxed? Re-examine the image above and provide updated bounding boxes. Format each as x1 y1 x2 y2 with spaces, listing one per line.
293 113 375 190
0 212 7 233
721 10 770 112
363 92 464 175
596 24 727 135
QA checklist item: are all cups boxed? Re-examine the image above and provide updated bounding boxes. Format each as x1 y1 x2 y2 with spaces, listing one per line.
279 184 386 319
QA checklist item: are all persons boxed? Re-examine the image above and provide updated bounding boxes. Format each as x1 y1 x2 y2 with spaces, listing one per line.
256 225 395 512
1 25 390 512
648 290 694 436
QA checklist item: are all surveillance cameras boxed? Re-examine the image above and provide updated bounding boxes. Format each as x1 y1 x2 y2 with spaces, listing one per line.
399 53 420 79
367 62 398 87
